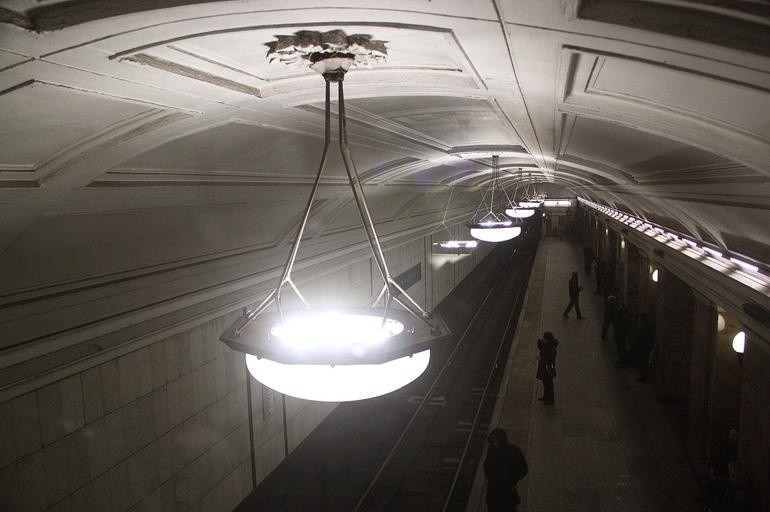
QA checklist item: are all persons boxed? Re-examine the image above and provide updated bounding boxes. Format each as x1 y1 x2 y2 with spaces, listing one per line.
599 263 616 305
481 427 529 511
601 286 624 341
563 270 586 321
633 313 653 384
704 426 739 512
535 332 560 407
717 460 762 512
581 244 594 274
611 304 629 370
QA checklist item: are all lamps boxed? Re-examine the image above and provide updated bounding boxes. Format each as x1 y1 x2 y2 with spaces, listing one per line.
730 329 745 366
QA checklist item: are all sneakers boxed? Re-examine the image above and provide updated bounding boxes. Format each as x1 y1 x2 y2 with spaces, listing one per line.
563 312 570 318
577 317 584 320
537 397 555 405
